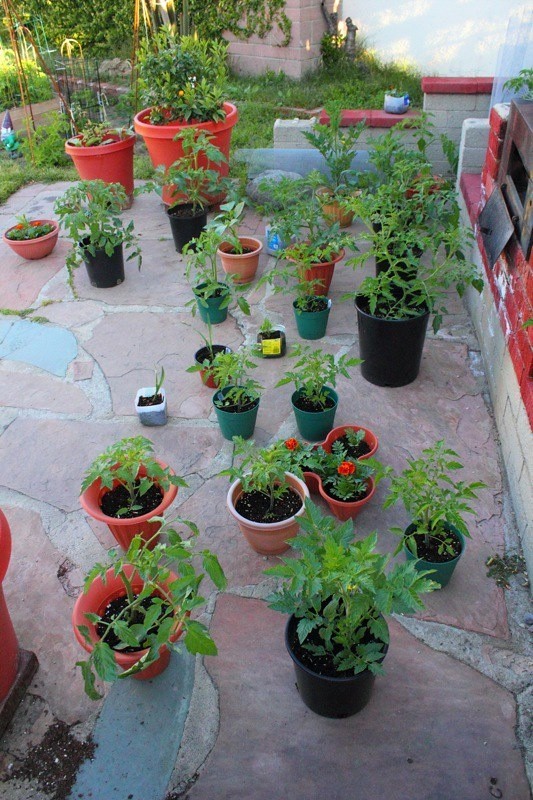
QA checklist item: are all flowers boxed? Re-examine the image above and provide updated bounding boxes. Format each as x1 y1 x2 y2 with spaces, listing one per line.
6 214 54 240
272 428 374 501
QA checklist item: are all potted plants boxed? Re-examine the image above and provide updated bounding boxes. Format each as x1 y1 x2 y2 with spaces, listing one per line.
340 183 484 387
71 513 227 701
261 500 443 720
134 365 168 427
186 341 268 440
366 437 488 592
181 312 232 388
58 25 461 323
272 344 366 442
81 437 188 555
220 438 310 556
255 317 285 358
258 266 334 339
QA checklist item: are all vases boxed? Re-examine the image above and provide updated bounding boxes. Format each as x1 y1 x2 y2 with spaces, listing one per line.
268 426 377 520
3 220 60 260
